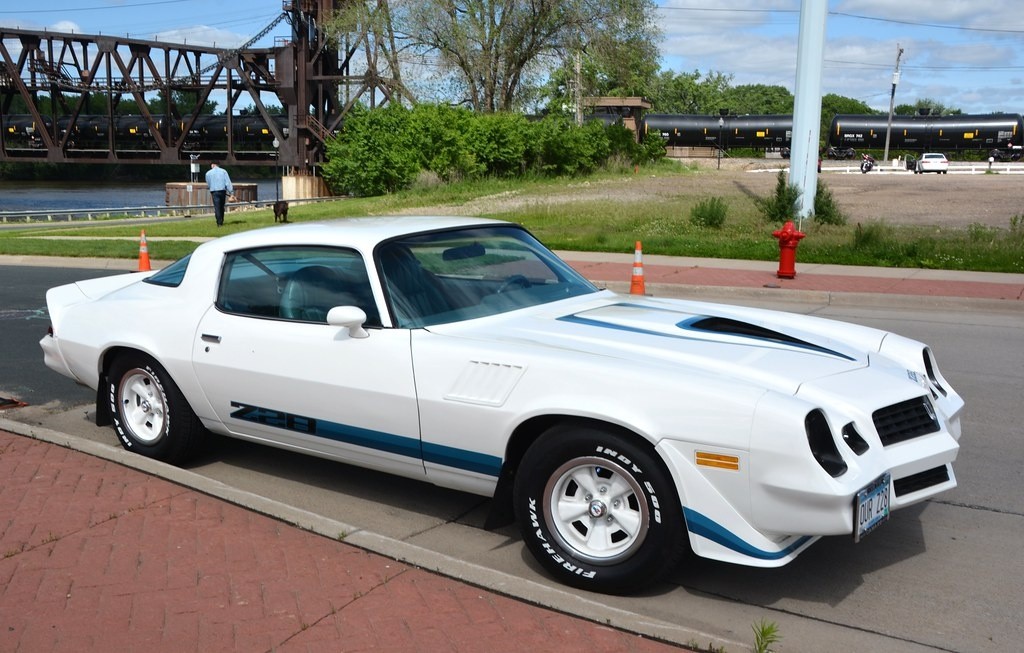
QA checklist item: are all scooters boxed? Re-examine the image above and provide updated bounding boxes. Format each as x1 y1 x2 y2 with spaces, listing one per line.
859 152 876 172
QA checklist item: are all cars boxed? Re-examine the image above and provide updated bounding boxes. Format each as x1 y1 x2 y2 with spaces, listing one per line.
827 147 857 160
903 153 949 175
39 217 966 599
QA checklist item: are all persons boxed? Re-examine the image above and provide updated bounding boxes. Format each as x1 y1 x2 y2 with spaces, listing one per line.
205 160 234 227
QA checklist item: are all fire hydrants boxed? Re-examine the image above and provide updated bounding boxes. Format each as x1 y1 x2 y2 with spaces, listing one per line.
772 222 810 283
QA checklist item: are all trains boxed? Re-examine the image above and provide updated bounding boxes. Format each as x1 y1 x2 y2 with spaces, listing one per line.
517 96 1024 162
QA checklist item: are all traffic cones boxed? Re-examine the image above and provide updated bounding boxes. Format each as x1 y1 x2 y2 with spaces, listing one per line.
630 239 654 296
128 229 155 274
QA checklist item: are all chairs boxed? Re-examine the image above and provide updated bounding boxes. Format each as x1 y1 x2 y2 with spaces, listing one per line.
382 246 461 315
278 264 376 330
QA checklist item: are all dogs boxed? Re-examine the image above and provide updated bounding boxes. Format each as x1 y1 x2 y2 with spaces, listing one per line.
273 200 289 224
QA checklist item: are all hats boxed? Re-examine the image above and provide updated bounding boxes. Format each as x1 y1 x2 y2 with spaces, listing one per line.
211 160 219 164
